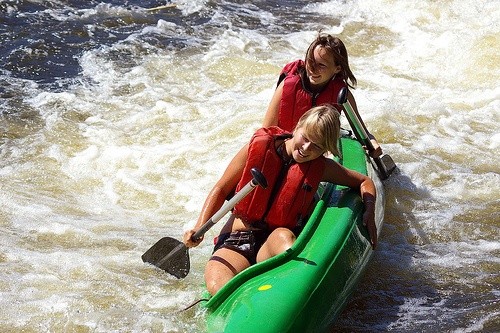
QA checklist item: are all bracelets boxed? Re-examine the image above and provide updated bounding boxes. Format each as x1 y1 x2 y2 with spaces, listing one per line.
364 199 375 204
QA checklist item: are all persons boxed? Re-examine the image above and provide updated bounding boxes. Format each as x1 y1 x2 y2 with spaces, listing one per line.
264 32 382 160
181 105 379 297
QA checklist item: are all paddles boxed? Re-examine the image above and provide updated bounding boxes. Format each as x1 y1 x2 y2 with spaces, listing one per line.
140 165 271 281
336 86 401 181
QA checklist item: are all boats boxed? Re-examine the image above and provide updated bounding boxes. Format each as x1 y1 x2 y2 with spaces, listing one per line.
198 132 387 333
